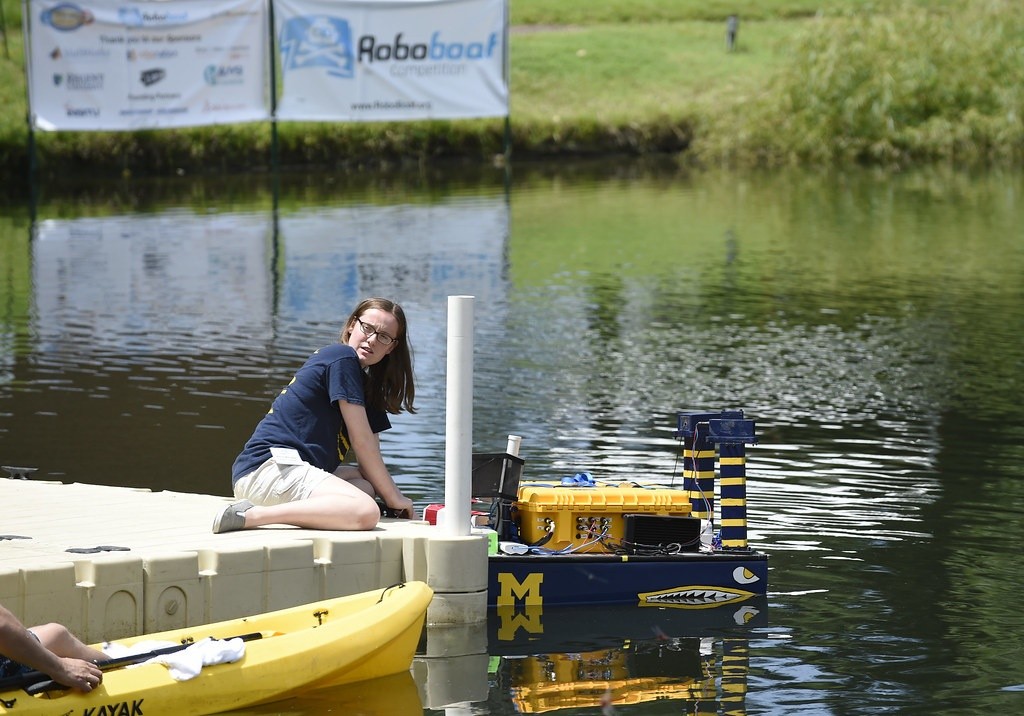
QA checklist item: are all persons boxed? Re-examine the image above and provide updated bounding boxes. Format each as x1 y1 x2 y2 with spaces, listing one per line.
212 298 419 535
0 604 128 694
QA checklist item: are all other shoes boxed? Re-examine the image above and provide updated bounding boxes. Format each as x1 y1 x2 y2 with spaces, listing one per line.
212 499 253 534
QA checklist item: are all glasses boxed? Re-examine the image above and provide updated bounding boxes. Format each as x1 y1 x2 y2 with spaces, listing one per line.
356 316 398 345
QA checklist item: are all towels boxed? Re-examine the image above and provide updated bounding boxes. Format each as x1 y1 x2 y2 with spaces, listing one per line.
104 637 246 682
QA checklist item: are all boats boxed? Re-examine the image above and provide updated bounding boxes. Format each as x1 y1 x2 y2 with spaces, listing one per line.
0 579 434 715
